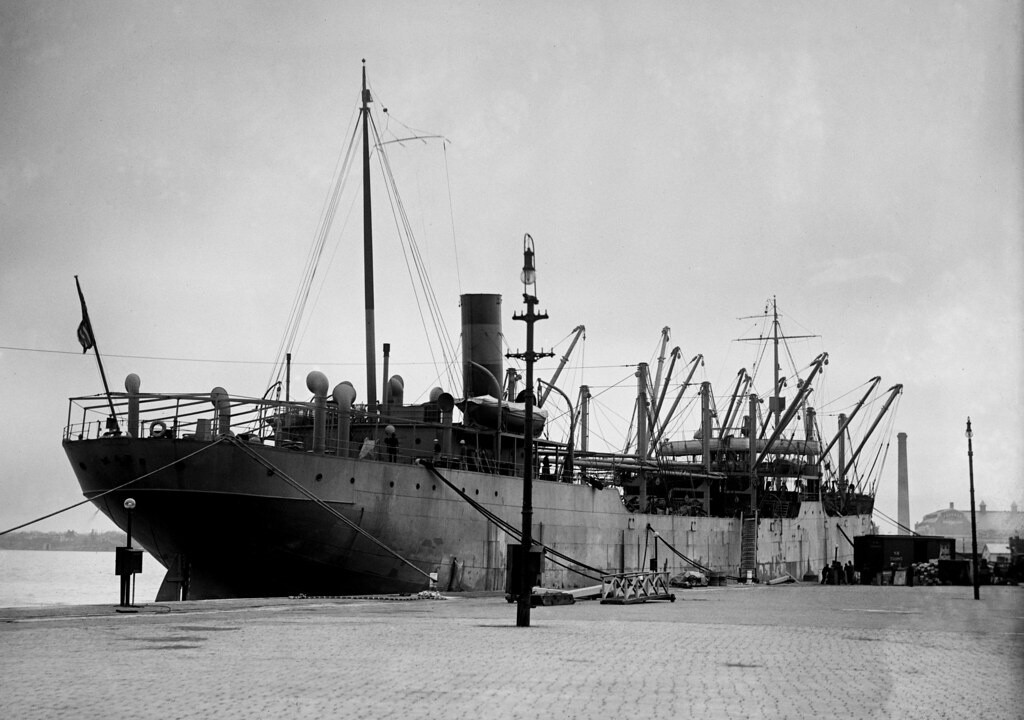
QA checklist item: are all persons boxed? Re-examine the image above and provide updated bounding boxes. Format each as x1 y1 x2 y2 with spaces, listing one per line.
384 433 400 462
992 562 1018 585
907 563 915 586
980 559 991 584
781 481 787 491
821 561 854 585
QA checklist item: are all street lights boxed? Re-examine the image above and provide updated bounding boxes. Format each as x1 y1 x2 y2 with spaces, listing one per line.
516 232 556 628
965 416 980 600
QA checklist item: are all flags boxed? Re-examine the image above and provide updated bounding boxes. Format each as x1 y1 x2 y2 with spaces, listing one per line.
824 463 830 470
76 286 94 353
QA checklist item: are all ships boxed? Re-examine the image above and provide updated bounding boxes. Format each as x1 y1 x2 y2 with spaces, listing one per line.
62 57 904 597
